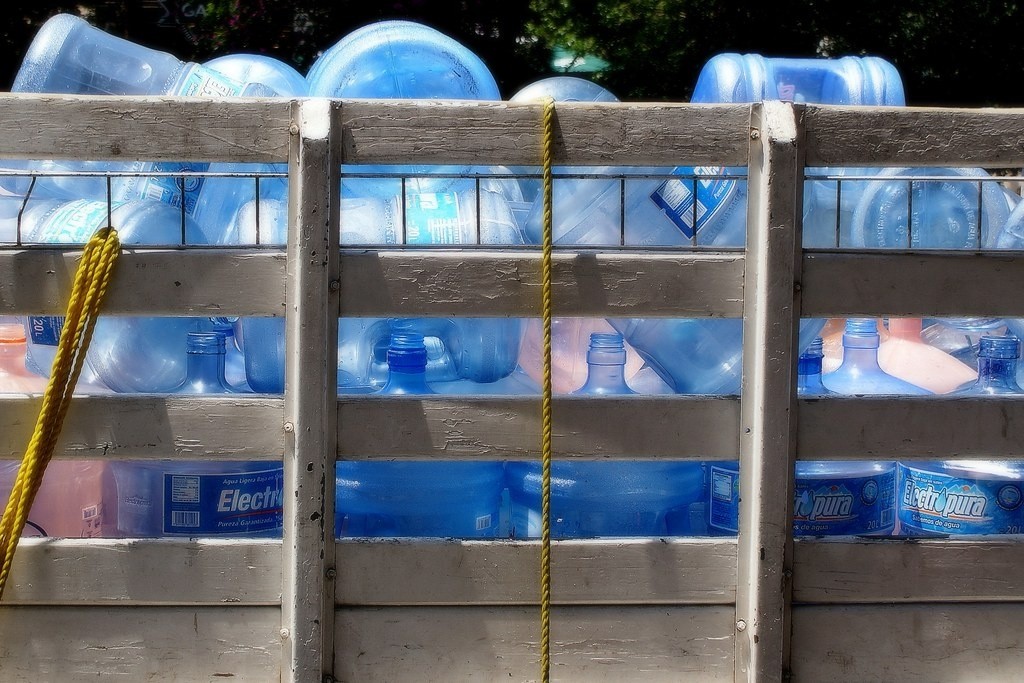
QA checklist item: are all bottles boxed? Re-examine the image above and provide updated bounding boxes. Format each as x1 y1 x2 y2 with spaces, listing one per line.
0 14 1023 543
